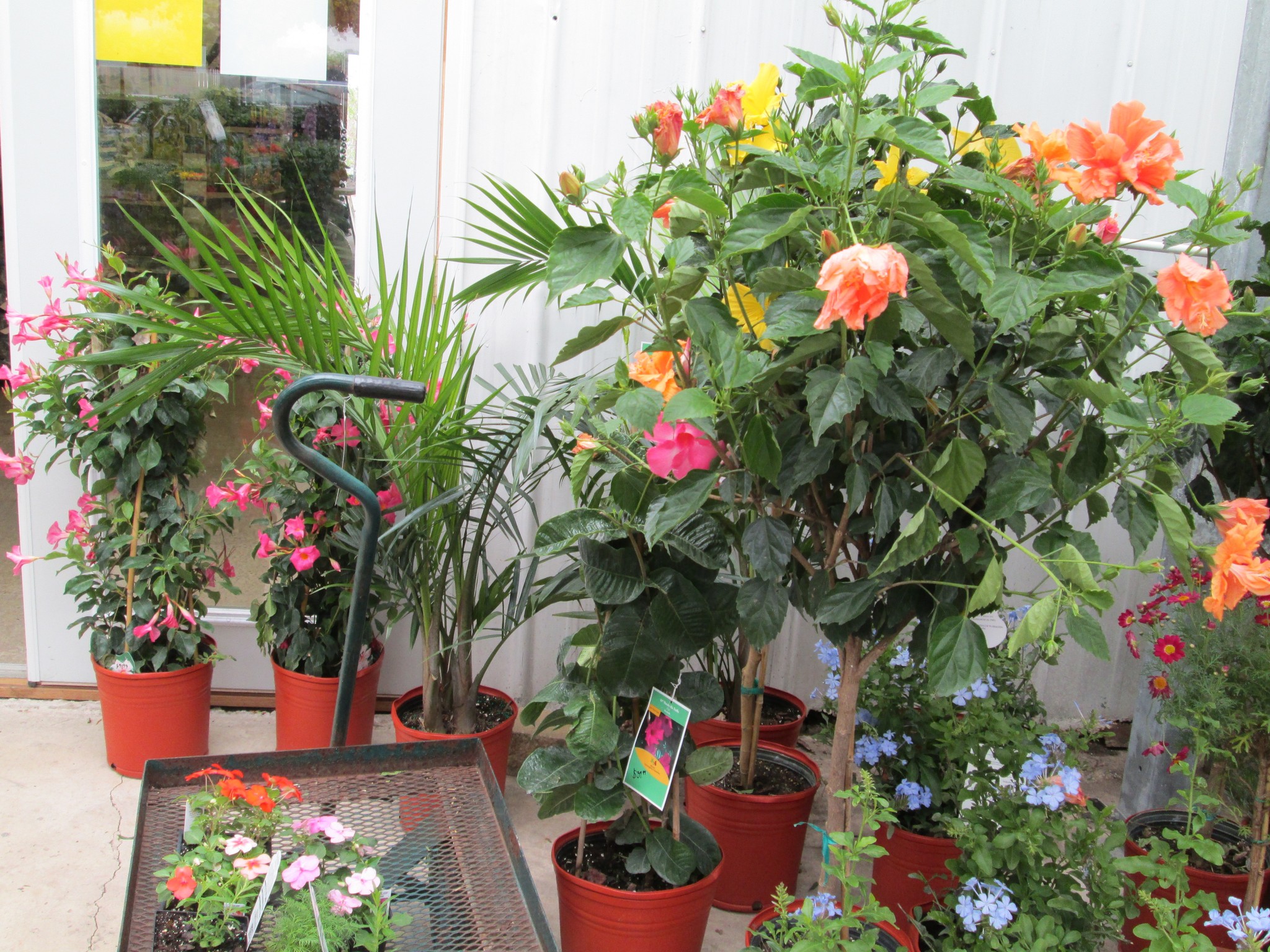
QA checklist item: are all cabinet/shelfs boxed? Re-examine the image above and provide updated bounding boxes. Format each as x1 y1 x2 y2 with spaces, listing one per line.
99 127 291 299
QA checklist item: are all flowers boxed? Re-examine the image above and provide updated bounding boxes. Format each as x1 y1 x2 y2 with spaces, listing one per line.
0 242 446 683
153 759 413 952
436 0 1270 952
97 89 349 203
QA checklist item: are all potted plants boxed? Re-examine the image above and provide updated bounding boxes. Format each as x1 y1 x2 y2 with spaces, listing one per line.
52 153 631 829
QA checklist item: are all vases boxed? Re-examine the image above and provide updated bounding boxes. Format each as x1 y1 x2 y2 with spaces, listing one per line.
92 632 219 778
685 687 808 748
270 631 385 801
553 817 723 952
1121 809 1270 952
871 814 963 933
683 737 822 913
746 898 916 952
